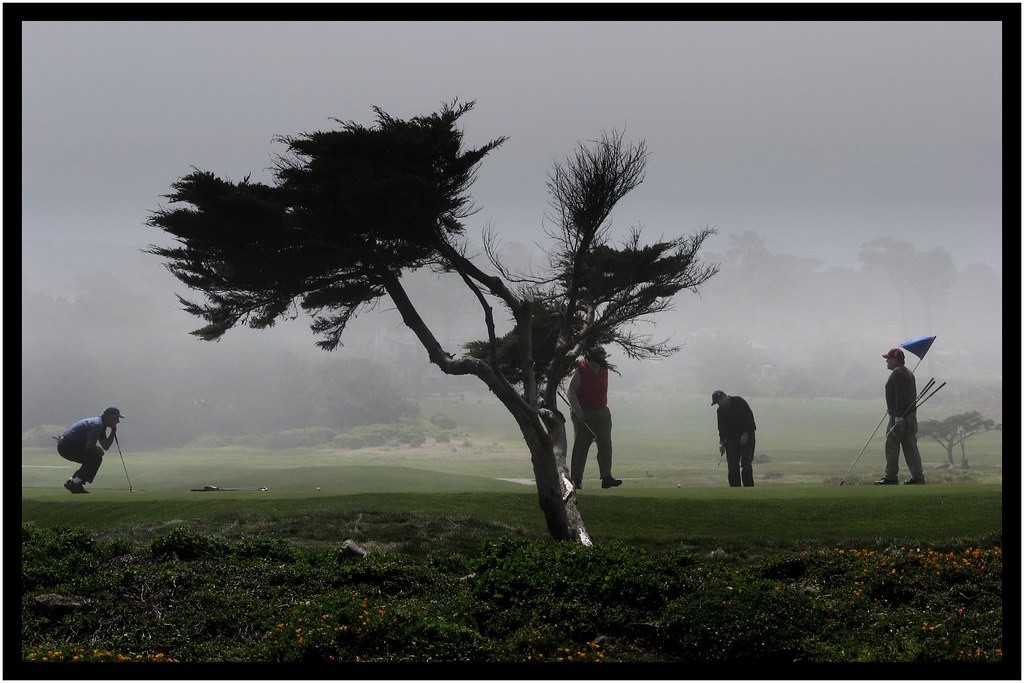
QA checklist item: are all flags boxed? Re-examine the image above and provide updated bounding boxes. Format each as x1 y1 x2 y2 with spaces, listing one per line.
898 336 935 360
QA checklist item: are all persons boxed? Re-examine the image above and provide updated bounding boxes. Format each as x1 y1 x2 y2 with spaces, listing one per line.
52 407 125 494
873 349 925 485
567 347 622 489
711 390 757 487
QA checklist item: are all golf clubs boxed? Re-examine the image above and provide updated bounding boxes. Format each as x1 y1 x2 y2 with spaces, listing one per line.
111 427 137 494
190 484 269 491
885 378 946 442
713 448 726 486
557 391 600 443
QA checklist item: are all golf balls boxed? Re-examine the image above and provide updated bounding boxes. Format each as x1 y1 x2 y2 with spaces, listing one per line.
678 484 682 488
317 487 321 491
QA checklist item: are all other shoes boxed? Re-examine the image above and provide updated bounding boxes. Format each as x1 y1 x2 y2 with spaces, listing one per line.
64 479 71 488
68 482 90 493
903 478 925 485
575 485 582 489
602 478 623 488
873 478 899 485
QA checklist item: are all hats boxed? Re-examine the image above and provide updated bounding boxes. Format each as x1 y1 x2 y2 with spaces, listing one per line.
595 347 606 354
711 391 725 406
882 349 905 362
103 407 125 418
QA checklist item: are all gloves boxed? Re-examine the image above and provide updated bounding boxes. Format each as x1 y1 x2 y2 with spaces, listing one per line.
895 417 904 425
